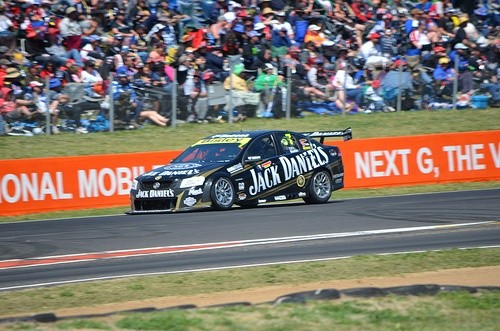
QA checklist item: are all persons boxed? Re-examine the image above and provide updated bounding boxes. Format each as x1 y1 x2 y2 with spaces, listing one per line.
0 0 500 137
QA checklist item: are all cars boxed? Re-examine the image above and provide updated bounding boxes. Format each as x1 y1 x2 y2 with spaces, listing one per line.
124 127 353 215
